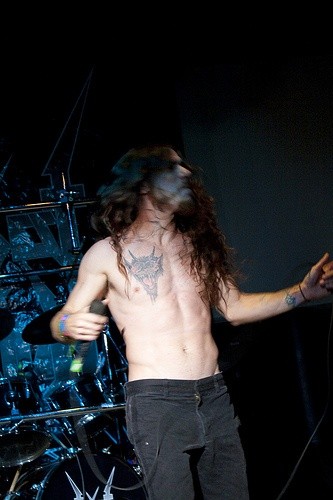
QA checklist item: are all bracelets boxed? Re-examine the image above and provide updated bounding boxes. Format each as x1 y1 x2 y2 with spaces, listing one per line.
298 283 308 303
57 313 69 339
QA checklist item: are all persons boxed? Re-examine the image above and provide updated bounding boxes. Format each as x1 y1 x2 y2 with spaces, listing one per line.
48 140 332 500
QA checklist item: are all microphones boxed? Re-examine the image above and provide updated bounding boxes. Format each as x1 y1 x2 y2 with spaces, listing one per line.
69 299 105 372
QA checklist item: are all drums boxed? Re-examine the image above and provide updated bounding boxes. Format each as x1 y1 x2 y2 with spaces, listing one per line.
0 376 53 469
41 374 115 435
6 446 150 500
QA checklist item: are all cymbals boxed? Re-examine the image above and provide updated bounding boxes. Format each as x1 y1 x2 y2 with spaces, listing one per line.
0 307 15 342
21 304 65 345
